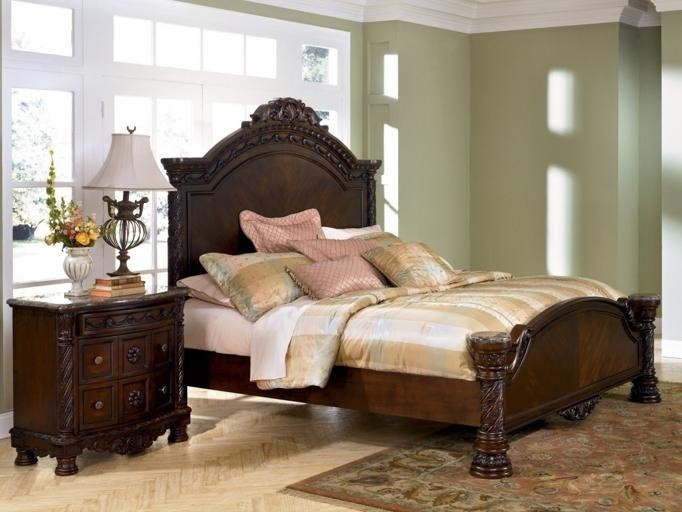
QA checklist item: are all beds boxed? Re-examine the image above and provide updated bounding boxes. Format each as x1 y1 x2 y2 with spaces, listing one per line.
158 98 666 479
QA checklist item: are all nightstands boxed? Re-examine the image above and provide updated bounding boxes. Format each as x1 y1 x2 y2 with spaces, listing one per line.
4 285 195 475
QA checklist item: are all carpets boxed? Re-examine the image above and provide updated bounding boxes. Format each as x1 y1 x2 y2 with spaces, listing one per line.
285 375 682 509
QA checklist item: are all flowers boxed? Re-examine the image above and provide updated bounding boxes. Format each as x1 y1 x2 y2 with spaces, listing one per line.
43 149 102 249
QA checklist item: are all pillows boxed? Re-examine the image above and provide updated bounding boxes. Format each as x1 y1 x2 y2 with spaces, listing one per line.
175 204 464 322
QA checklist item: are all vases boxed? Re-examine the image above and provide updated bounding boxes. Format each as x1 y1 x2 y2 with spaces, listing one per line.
63 246 95 298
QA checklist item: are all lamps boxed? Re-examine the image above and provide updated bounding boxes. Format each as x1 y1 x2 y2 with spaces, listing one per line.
79 127 182 277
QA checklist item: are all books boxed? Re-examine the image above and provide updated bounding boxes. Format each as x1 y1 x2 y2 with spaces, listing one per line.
88 275 146 298
